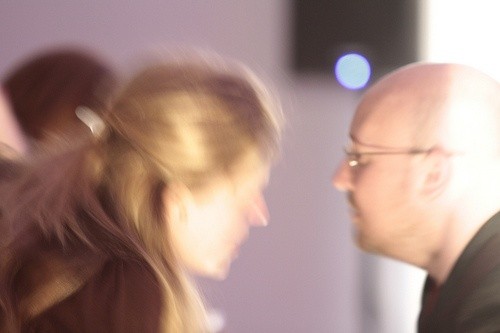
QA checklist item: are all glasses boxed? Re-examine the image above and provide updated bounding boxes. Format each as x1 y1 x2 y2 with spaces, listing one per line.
336 124 449 166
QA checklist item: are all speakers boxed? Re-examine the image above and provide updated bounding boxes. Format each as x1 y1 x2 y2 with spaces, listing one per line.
289 0 420 76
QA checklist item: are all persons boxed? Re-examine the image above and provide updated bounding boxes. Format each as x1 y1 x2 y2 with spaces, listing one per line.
0 45 285 333
0 46 125 164
331 60 500 333
